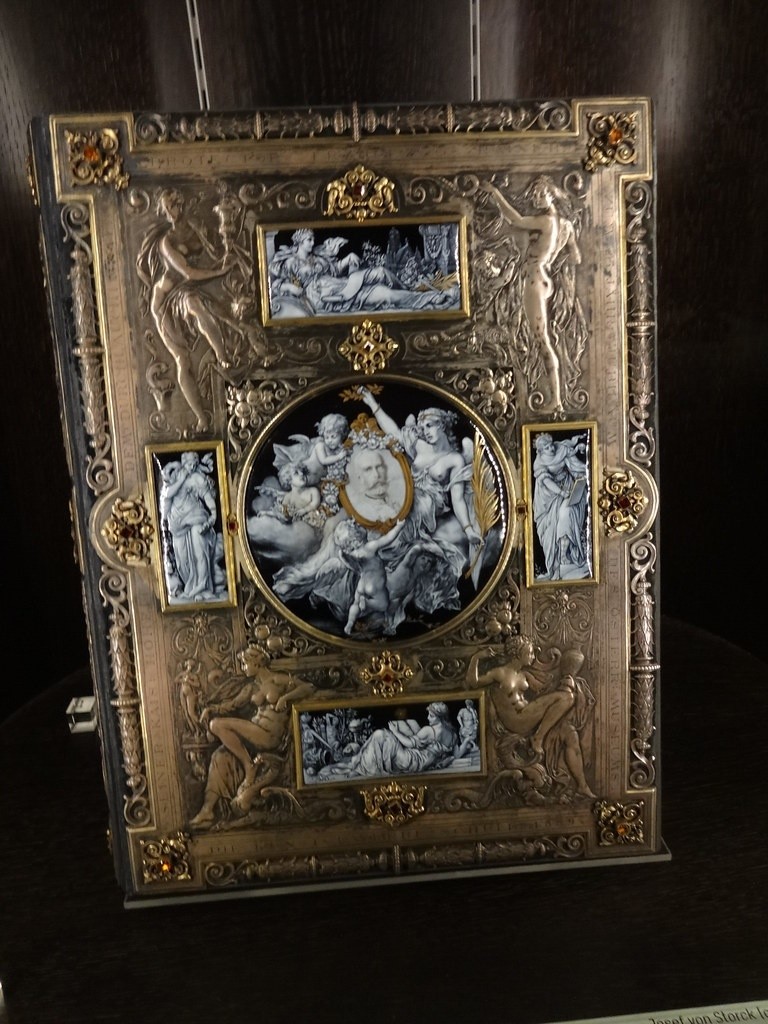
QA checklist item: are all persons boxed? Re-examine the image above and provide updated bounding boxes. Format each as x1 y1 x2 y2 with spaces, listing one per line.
135 164 613 833
353 450 401 521
